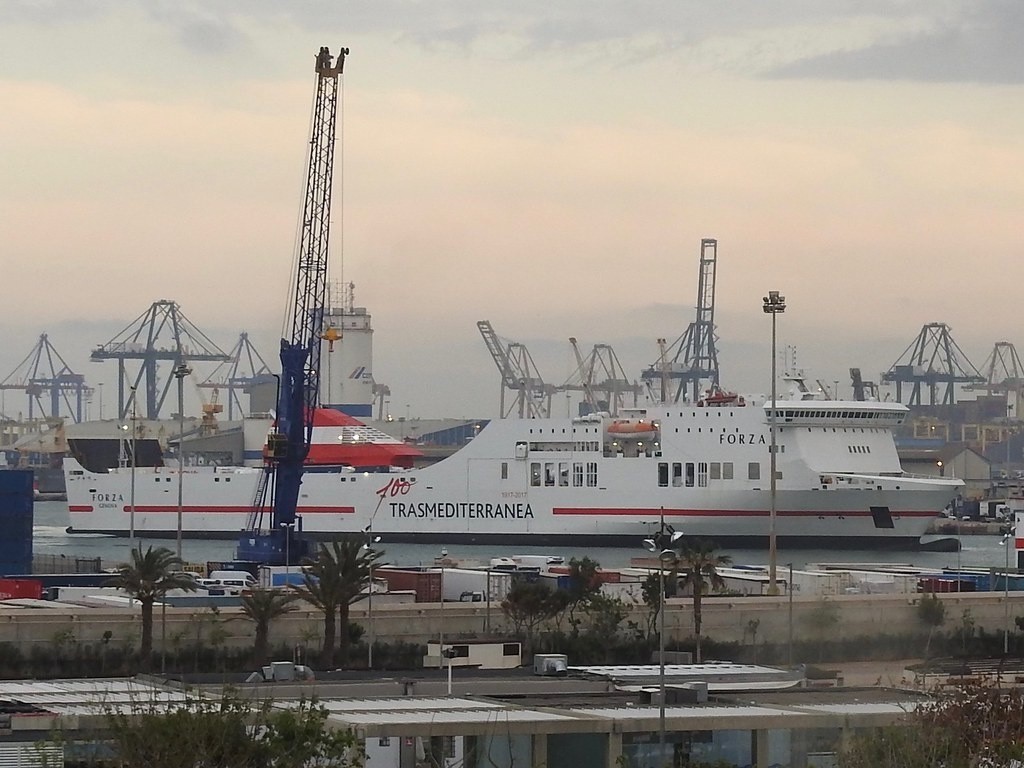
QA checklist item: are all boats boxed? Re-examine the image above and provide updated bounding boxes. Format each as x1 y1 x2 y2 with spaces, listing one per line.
605 420 656 443
703 391 739 404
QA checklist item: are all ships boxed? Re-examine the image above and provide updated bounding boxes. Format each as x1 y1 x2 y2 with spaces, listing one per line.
61 387 968 547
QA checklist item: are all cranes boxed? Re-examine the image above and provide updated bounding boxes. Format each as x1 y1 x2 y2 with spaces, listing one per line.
477 238 720 418
0 299 274 449
883 321 1024 407
233 44 352 560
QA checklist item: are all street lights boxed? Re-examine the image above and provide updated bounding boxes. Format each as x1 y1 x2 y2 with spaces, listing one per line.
640 500 685 768
280 522 297 615
996 518 1018 654
761 287 788 594
